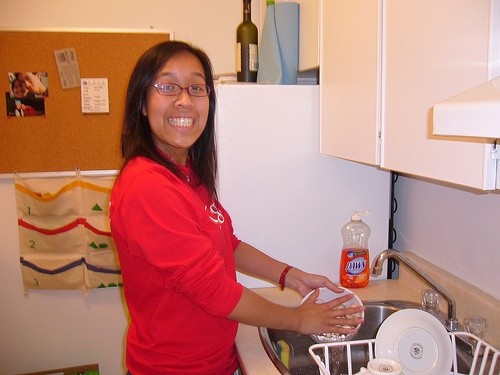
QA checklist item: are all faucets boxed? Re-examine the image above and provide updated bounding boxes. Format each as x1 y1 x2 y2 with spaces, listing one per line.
372 248 460 343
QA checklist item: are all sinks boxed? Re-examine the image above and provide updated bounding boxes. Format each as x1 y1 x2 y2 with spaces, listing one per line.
251 297 424 365
282 345 496 375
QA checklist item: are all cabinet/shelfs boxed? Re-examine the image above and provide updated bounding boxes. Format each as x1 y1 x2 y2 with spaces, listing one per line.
256 0 500 193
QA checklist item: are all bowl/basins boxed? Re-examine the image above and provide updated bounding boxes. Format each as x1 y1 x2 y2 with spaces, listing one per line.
299 286 364 345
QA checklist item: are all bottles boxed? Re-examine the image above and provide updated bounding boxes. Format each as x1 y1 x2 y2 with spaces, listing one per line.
340 210 372 289
236 0 258 83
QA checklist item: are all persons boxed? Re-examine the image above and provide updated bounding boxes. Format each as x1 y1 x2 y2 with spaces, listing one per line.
109 41 365 375
12 72 48 97
14 100 35 116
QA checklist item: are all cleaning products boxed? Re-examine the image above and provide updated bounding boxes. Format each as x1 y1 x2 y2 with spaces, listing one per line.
336 211 374 293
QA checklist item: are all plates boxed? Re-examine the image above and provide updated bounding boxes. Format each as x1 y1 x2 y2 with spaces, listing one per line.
367 359 403 375
374 308 453 375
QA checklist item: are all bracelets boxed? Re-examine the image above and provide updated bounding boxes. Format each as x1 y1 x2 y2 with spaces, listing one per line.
279 266 292 287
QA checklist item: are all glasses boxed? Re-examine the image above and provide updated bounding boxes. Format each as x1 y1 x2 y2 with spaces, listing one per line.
146 83 210 97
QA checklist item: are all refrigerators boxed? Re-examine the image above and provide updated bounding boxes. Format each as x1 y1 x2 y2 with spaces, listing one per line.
210 78 398 289
